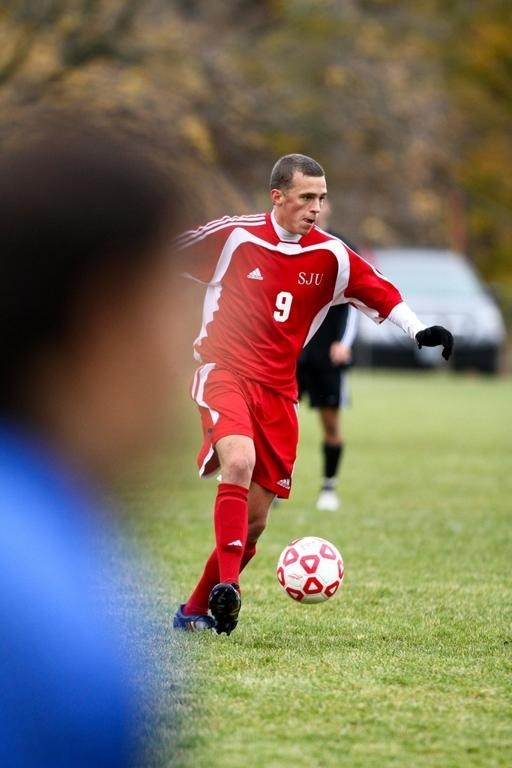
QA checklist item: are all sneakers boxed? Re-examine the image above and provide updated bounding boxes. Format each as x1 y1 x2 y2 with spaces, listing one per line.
315 489 340 513
172 583 240 638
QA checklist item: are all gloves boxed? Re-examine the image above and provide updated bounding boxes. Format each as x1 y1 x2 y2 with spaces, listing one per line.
415 324 455 360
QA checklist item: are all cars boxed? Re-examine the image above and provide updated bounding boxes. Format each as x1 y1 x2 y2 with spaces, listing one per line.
353 246 506 374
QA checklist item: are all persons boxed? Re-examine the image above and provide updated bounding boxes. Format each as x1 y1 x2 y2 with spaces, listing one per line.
171 154 454 633
289 197 361 512
4 83 230 766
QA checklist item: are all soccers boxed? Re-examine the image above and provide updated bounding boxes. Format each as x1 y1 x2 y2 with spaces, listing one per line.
277 537 344 603
